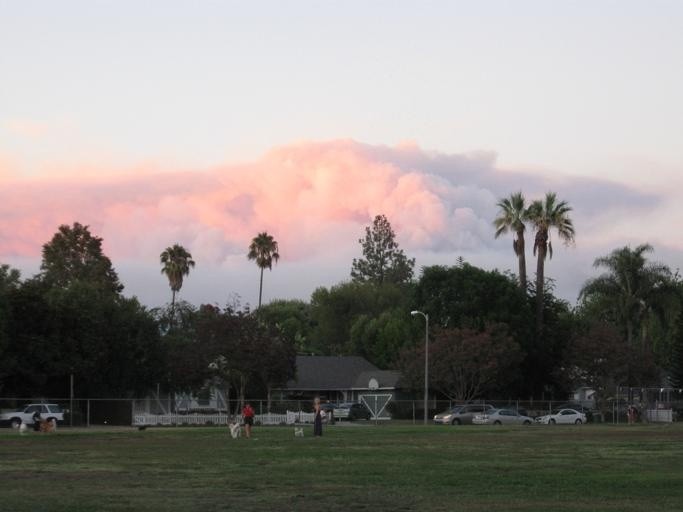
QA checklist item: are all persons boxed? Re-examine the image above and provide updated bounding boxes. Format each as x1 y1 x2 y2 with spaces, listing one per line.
626 405 634 424
241 403 255 438
312 397 322 436
33 411 42 432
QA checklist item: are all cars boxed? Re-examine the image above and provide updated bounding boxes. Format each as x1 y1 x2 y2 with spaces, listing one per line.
433 404 587 425
0 403 65 429
318 402 372 421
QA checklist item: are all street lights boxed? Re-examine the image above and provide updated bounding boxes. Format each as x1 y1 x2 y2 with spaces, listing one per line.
411 310 429 425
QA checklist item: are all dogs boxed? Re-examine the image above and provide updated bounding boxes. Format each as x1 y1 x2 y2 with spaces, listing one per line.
229 422 242 438
294 427 304 438
40 421 55 433
